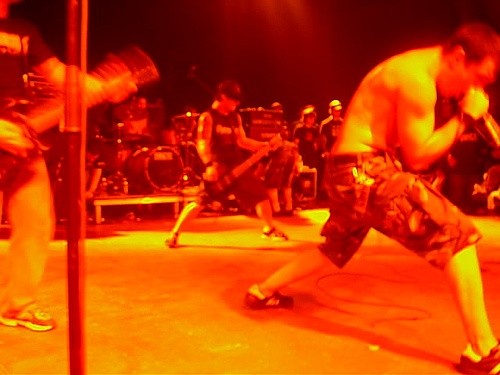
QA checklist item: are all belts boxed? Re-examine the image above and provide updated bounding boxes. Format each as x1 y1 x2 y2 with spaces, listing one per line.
323 151 383 163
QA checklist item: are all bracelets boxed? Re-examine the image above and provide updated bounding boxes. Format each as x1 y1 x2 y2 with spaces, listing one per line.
455 107 477 128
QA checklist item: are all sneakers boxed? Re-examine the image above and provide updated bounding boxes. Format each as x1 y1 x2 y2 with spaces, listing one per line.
165 237 180 249
261 227 288 241
0 304 57 331
245 283 292 309
461 340 500 375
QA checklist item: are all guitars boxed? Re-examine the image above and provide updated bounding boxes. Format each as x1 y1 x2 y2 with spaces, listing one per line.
202 134 286 201
0 43 163 195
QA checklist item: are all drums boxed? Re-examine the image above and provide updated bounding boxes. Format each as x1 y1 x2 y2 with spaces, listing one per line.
127 145 187 193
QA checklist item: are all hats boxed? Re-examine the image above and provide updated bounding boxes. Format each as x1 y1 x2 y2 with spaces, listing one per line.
329 99 342 114
217 78 243 101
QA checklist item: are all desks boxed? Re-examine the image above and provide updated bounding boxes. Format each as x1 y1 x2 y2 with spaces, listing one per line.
93 196 182 226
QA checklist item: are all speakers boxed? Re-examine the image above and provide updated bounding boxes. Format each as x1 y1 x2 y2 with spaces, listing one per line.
240 108 284 142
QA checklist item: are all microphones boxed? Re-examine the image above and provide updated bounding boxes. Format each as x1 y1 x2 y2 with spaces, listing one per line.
480 114 500 149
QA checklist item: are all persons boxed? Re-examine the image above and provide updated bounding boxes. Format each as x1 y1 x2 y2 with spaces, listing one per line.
174 99 500 211
245 21 500 375
1 0 139 332
164 80 290 248
